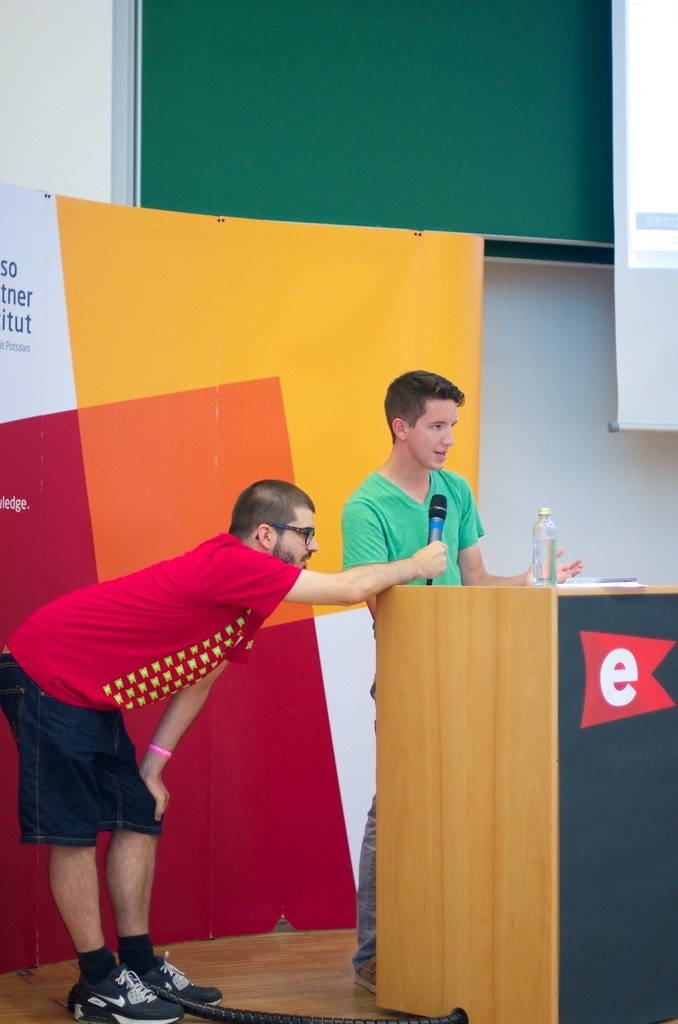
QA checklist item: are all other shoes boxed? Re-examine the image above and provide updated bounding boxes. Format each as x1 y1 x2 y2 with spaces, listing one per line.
355 953 376 993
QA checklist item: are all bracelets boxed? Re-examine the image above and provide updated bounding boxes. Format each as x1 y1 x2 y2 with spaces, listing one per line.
146 740 172 760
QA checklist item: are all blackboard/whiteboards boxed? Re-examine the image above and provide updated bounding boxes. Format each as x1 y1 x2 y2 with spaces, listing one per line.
115 0 613 266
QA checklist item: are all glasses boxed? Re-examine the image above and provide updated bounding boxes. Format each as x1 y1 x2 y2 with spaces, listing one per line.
255 522 315 545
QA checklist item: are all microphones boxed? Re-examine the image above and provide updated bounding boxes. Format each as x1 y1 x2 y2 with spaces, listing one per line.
427 494 447 584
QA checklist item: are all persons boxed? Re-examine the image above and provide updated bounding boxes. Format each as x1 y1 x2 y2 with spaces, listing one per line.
337 370 587 994
0 481 450 1024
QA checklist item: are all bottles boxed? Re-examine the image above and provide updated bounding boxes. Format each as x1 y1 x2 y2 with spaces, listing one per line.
532 508 558 586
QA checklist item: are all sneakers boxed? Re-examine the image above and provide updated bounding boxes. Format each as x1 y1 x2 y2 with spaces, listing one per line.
135 955 222 1006
67 963 184 1024
